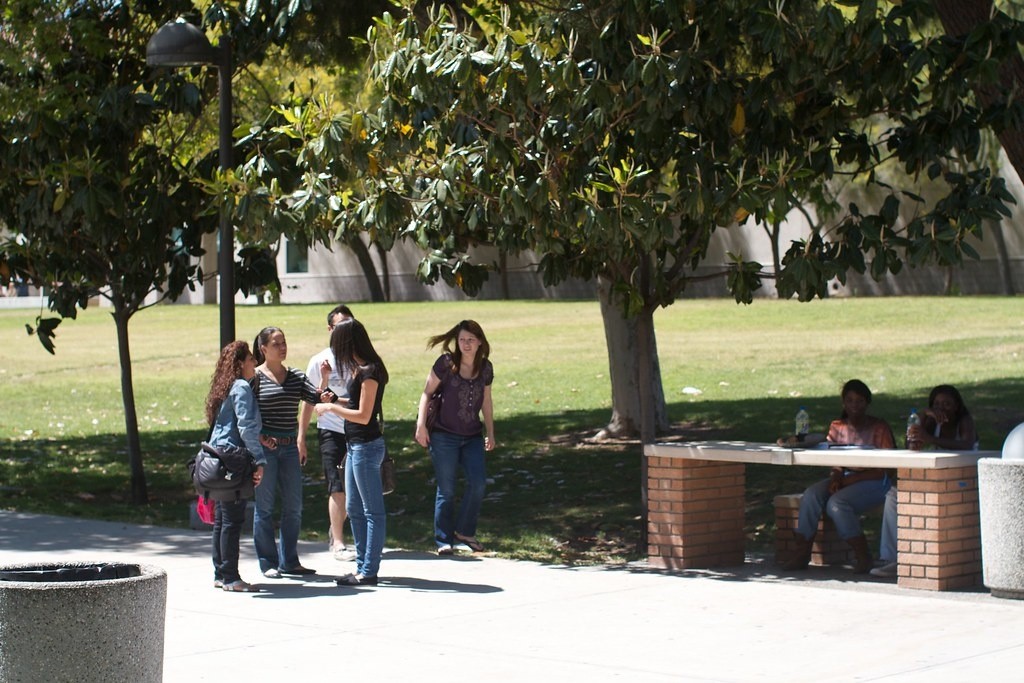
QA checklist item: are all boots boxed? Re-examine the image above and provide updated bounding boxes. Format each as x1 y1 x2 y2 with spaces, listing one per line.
783 531 817 570
848 533 872 573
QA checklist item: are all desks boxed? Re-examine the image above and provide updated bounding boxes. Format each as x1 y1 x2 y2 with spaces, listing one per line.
644 442 1001 594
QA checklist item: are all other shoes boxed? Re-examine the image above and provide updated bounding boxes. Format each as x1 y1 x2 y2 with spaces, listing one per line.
263 569 281 578
333 545 356 561
277 563 316 574
329 526 333 551
337 576 378 585
870 562 897 577
333 573 360 583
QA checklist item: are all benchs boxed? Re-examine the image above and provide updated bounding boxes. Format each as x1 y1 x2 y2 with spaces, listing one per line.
773 494 883 570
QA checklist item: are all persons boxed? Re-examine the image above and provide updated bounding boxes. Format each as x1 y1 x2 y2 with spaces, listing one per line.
246 328 337 578
779 376 899 575
869 385 979 575
213 342 270 593
413 319 496 556
297 304 390 586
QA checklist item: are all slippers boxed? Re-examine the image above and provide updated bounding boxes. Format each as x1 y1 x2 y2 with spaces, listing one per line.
454 533 484 552
438 544 452 555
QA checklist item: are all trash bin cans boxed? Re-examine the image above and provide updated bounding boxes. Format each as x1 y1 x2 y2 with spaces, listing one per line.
1 559 167 682
977 424 1023 599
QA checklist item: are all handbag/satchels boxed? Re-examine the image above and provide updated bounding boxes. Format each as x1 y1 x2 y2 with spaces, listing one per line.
337 455 394 495
197 495 215 526
414 353 452 440
187 441 251 489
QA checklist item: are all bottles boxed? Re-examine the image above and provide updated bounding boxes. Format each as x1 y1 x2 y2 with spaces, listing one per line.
795 406 809 442
906 408 923 450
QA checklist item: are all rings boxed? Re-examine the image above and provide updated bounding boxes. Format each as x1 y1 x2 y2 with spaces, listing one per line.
259 475 260 479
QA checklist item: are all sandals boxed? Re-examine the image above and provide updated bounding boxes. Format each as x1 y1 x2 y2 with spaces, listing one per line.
215 579 224 587
222 580 259 592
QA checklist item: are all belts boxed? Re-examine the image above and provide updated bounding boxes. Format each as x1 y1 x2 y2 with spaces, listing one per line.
265 436 296 445
